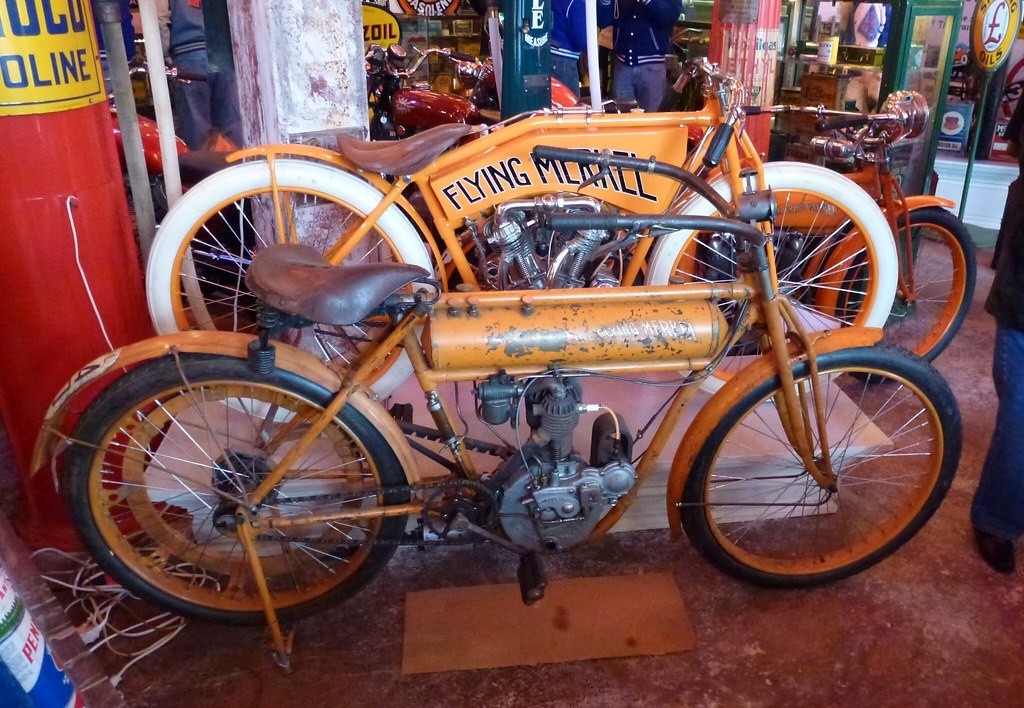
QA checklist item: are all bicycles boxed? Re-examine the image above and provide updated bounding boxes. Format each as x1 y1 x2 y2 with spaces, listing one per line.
27 49 979 681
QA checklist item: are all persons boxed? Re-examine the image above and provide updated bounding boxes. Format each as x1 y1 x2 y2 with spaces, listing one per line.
551 0 683 113
970 89 1024 574
90 0 243 151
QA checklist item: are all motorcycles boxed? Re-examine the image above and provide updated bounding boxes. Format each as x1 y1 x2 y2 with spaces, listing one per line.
356 34 514 134
